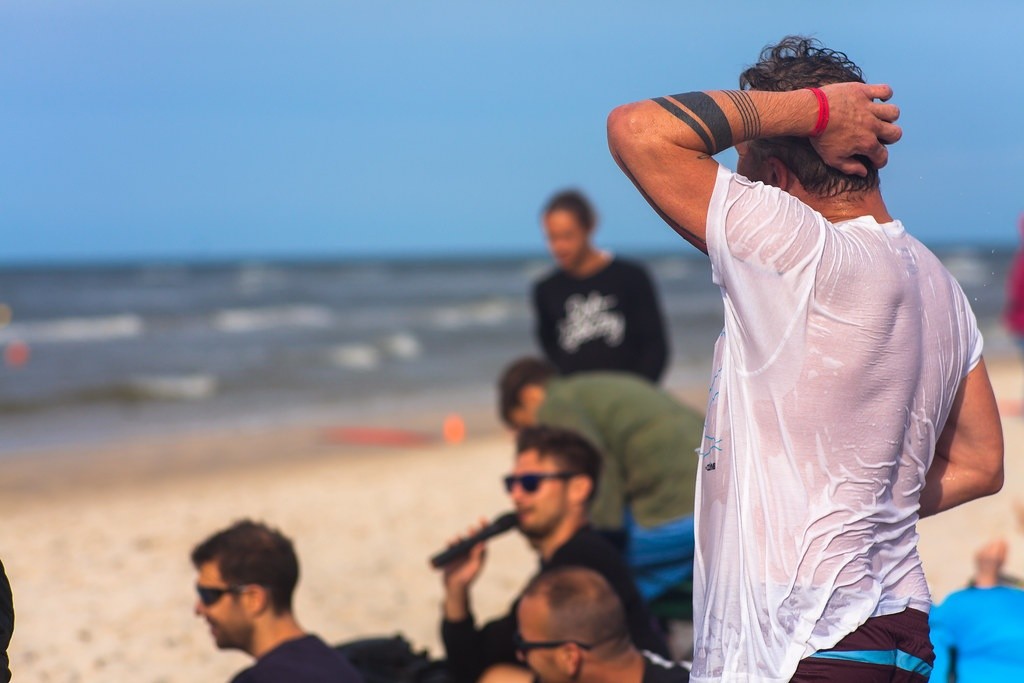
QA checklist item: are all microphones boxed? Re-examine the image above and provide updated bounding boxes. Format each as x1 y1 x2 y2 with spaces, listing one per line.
431 514 520 568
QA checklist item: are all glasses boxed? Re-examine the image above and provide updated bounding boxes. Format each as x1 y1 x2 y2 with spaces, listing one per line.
197 585 235 606
516 631 592 654
505 473 569 492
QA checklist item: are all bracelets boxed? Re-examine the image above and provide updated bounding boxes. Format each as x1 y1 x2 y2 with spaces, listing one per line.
808 87 829 139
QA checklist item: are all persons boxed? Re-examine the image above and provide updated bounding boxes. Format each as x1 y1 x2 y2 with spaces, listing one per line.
499 357 706 683
530 191 673 386
438 426 672 683
190 519 371 683
608 37 1005 683
482 567 692 683
